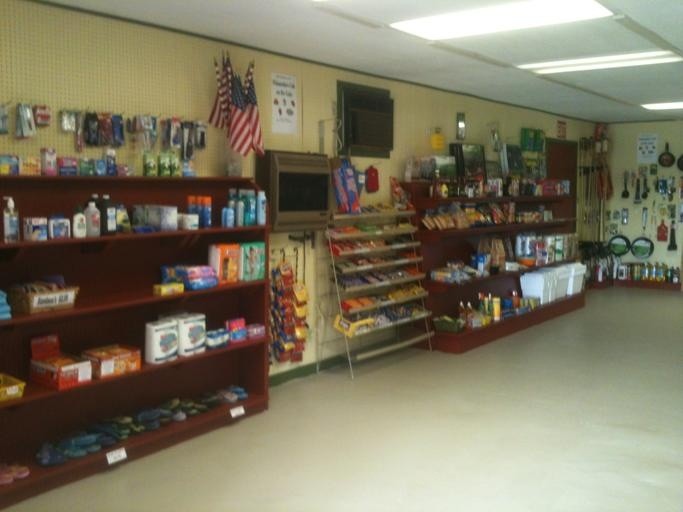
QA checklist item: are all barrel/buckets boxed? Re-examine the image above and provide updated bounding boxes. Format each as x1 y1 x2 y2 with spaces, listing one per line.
145 318 180 364
175 310 206 357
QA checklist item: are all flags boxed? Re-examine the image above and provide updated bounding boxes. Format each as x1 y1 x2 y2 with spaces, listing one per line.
206 48 265 161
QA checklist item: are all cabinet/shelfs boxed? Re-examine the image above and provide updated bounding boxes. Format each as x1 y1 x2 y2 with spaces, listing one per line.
1 175 271 510
409 174 586 355
316 202 436 379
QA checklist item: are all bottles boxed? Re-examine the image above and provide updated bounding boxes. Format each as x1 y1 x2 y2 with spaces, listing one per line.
187 196 213 228
85 194 116 238
256 190 266 226
